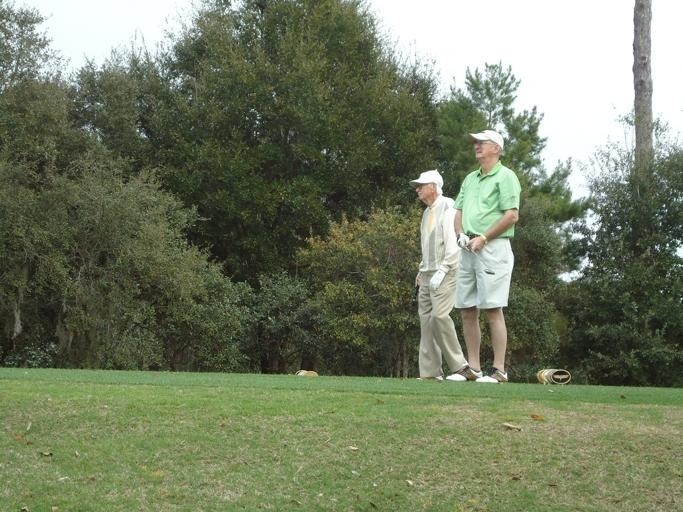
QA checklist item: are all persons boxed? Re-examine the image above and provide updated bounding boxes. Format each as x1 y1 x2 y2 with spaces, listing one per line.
445 130 522 383
408 169 468 381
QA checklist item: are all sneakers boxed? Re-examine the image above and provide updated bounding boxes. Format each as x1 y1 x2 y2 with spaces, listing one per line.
475 367 508 383
444 364 483 381
415 375 444 381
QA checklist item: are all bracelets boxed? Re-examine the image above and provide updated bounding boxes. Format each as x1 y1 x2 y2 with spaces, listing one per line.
480 234 488 245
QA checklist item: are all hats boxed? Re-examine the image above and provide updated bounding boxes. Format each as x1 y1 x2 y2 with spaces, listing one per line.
409 169 444 188
464 130 505 151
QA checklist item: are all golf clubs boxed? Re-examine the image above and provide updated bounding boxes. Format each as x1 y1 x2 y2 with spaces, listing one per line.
466 246 494 275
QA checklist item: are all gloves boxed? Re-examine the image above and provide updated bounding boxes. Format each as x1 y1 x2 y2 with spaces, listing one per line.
429 266 448 291
456 232 470 249
